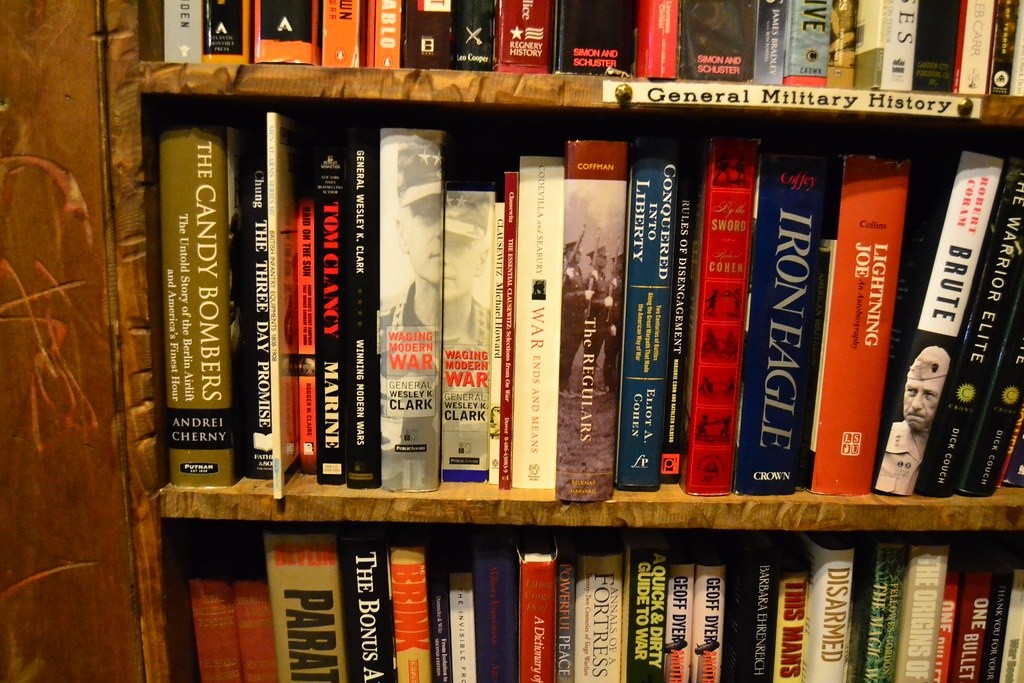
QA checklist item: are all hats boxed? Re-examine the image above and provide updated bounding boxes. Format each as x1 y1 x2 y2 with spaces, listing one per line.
586 245 610 259
611 253 624 263
445 191 489 240
908 347 951 380
396 146 442 207
565 241 581 250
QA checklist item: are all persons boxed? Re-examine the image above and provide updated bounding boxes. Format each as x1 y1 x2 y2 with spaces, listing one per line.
875 345 950 495
380 137 443 453
558 242 625 399
443 191 493 431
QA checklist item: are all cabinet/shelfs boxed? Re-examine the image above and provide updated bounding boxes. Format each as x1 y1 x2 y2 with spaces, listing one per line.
0 1 1024 682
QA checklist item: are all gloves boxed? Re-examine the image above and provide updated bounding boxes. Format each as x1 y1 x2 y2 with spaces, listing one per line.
604 296 613 307
591 269 598 281
585 290 594 300
611 277 617 289
566 267 575 279
610 325 617 336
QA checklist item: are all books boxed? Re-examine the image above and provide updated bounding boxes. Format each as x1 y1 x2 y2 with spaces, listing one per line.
164 0 1024 98
159 112 1023 503
188 533 1024 683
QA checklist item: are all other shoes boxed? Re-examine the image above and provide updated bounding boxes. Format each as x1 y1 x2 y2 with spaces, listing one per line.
559 388 582 399
577 385 606 398
605 385 619 393
